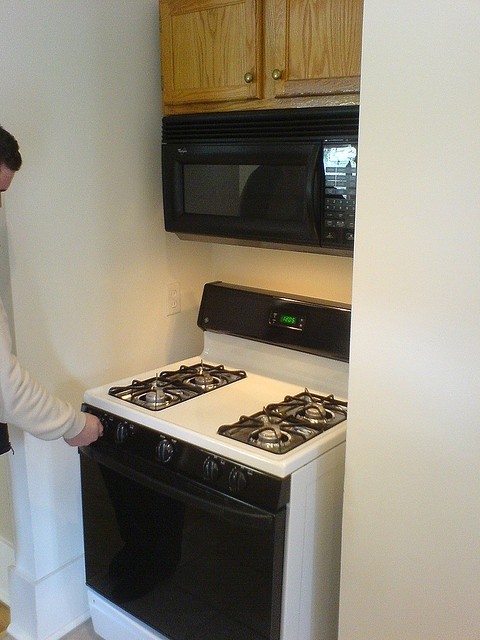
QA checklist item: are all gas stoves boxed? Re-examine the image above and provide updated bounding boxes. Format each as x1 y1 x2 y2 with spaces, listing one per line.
84 354 349 467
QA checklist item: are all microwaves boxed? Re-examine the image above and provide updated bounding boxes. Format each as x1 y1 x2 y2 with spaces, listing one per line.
158 106 360 254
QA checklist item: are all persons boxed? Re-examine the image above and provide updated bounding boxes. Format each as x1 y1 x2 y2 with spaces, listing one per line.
0 124 105 455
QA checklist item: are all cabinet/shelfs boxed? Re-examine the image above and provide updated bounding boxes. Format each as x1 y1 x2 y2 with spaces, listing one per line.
158 2 365 116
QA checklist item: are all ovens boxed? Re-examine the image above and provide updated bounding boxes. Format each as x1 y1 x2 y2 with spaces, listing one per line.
74 401 284 638
